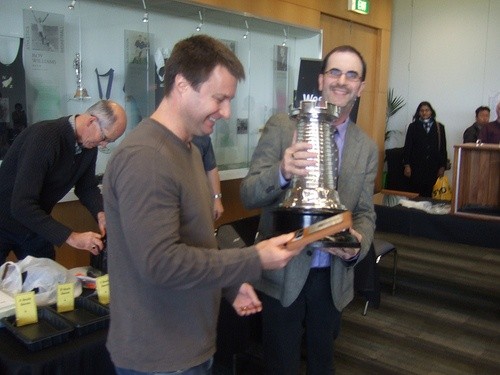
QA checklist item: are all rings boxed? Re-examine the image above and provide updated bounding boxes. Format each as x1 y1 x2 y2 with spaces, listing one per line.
291 152 295 159
91 245 96 250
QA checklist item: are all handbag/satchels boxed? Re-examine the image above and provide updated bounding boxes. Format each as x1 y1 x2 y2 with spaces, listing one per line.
432 175 452 201
437 121 451 170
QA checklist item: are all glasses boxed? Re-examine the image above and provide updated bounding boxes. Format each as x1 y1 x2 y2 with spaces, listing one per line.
90 114 112 143
323 68 363 82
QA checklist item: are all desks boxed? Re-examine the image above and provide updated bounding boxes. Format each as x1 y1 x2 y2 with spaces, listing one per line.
0 294 258 375
450 139 500 228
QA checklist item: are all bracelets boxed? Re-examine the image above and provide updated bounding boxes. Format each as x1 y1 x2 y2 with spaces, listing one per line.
211 192 224 200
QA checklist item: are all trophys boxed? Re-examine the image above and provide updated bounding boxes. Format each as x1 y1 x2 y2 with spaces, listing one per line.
73 52 87 97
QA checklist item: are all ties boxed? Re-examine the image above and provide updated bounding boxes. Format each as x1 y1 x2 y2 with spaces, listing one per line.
329 130 340 189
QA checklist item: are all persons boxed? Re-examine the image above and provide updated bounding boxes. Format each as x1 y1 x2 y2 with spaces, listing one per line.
402 102 448 197
477 103 500 145
0 100 127 267
104 36 306 375
239 46 377 375
462 106 490 144
191 131 225 220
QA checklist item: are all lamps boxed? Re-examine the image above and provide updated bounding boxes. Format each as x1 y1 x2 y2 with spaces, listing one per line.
72 52 94 100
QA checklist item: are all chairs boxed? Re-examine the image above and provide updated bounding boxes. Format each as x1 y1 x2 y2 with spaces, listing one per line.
354 233 403 316
